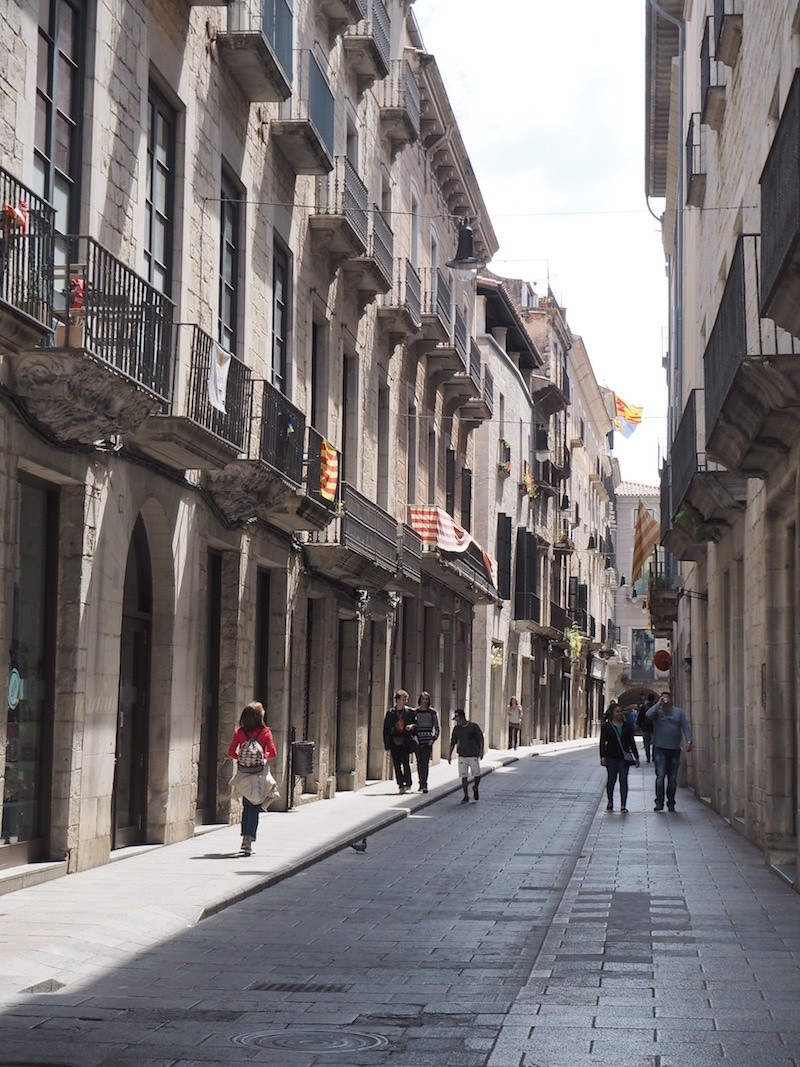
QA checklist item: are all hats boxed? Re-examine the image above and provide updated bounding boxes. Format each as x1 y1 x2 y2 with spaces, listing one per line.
451 709 464 720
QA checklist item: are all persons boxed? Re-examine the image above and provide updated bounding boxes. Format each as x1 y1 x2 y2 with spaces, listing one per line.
448 709 485 804
383 690 418 796
601 694 657 763
506 697 523 750
228 702 281 857
647 692 694 813
412 692 440 794
598 703 640 814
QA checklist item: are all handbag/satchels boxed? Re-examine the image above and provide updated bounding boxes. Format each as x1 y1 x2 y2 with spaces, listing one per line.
624 752 635 765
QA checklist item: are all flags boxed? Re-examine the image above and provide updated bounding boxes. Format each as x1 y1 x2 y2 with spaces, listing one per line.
611 395 643 430
628 497 660 588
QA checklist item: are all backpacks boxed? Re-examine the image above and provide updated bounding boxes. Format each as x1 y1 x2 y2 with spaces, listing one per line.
237 727 265 774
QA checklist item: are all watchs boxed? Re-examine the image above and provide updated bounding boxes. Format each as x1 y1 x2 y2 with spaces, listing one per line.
687 741 692 743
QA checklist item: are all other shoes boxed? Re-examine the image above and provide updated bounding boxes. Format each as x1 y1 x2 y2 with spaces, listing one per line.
514 746 516 750
472 786 479 800
400 788 405 794
656 802 663 810
508 747 512 749
607 801 613 811
462 797 469 802
621 807 628 813
404 786 411 790
668 803 674 812
241 842 256 856
418 787 428 793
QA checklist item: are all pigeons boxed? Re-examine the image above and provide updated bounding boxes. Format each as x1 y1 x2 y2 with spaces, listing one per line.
349 837 367 853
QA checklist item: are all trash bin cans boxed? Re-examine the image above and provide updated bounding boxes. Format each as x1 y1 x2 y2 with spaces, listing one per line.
291 740 315 776
